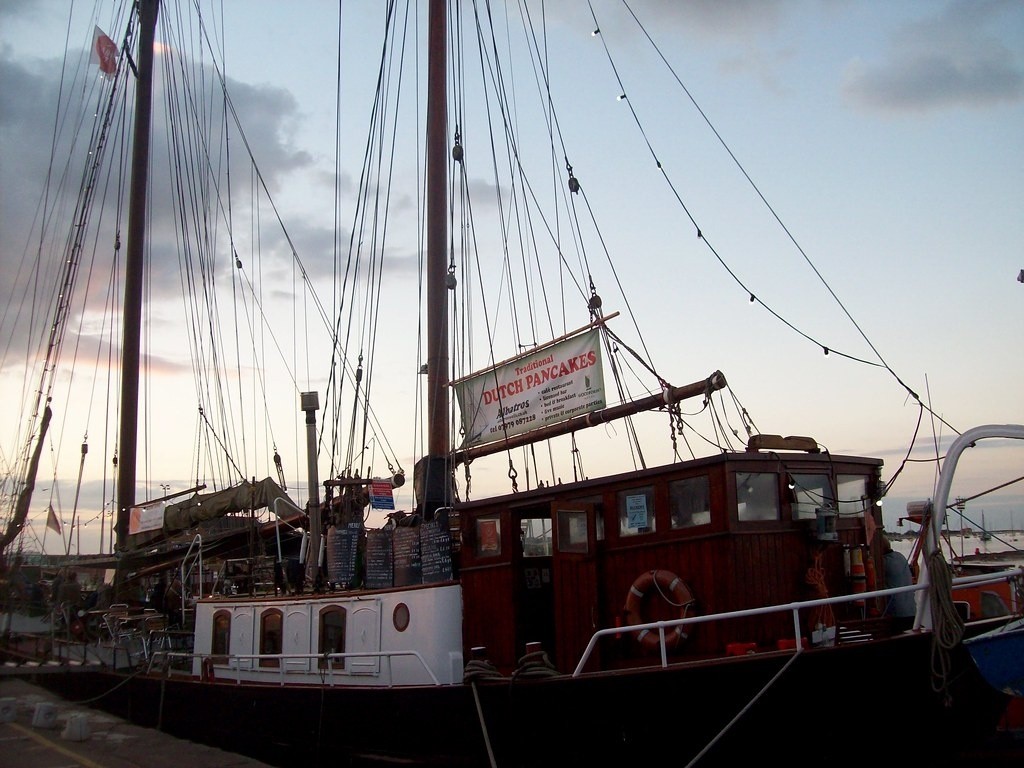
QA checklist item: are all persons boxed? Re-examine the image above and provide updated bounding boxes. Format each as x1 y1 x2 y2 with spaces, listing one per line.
671 498 694 529
51 568 80 601
325 629 341 659
883 539 917 637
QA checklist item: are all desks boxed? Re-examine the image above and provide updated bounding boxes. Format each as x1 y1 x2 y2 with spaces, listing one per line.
149 629 193 665
88 607 144 648
119 613 162 620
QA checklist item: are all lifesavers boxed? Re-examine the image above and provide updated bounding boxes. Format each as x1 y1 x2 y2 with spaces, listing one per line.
623 570 697 651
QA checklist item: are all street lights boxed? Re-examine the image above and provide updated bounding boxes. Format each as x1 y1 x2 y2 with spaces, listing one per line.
160 483 170 504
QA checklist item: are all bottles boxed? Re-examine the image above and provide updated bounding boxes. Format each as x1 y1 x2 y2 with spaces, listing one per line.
822 626 829 647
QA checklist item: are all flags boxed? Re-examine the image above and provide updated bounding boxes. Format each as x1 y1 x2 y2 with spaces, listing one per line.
46 506 61 535
89 26 119 81
127 500 165 535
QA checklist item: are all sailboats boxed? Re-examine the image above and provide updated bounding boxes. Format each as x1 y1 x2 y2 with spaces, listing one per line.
0 1 1024 767
978 509 992 540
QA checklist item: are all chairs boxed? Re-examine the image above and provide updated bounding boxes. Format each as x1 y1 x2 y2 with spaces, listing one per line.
96 604 172 666
